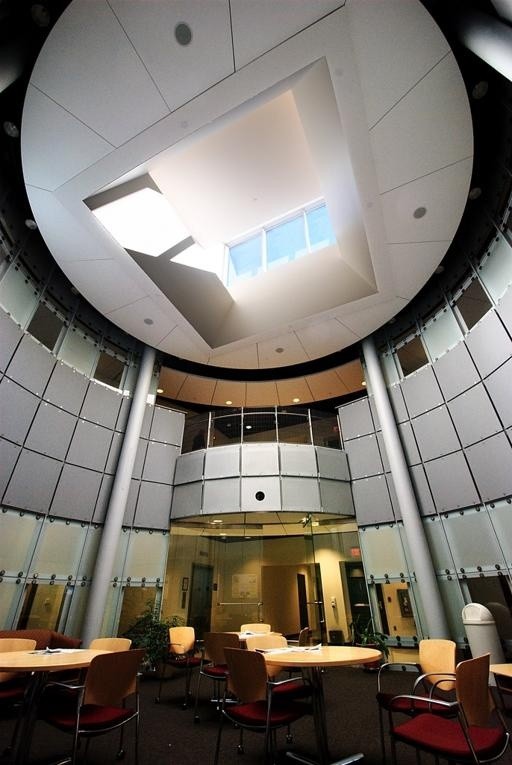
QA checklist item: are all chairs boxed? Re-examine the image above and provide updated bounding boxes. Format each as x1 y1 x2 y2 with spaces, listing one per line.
0 623 151 764
377 639 511 765
156 623 317 764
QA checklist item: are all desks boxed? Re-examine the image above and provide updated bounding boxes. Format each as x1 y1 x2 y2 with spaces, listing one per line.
0 648 115 764
257 645 382 765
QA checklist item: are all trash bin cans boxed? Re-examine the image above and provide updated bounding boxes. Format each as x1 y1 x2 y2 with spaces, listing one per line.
462 601 512 688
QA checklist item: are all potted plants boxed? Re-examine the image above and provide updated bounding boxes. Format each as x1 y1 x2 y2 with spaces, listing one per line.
351 615 389 670
121 598 176 681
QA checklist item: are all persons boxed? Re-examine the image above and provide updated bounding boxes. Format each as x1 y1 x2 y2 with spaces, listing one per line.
328 424 342 449
191 429 206 450
